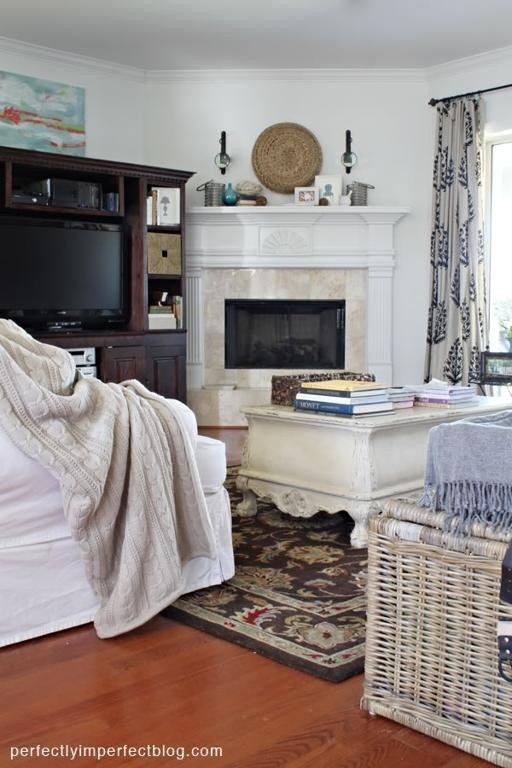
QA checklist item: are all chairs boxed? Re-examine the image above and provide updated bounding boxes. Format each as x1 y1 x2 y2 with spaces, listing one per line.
360 412 512 768
1 398 235 651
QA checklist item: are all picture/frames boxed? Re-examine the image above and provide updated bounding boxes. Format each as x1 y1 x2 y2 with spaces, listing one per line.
294 186 320 206
480 351 512 381
314 175 342 206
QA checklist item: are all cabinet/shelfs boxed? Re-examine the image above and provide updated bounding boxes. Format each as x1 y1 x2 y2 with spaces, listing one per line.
0 145 196 406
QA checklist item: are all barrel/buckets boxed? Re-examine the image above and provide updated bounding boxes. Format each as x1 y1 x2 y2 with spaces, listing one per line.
347 181 374 206
197 179 224 206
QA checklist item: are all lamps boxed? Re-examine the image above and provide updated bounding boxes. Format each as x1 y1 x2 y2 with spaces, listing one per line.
213 131 231 175
341 130 357 173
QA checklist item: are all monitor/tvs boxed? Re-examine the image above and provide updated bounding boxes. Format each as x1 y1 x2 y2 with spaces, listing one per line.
0 213 132 332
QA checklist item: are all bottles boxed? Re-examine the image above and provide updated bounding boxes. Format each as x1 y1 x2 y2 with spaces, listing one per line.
224 182 237 205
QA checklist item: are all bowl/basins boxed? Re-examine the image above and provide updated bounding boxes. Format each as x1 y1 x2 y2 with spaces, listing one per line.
235 190 261 200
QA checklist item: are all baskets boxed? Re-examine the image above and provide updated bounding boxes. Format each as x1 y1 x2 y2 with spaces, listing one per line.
363 500 512 768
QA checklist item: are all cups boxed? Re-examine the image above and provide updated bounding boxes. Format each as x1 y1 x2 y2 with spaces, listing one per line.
106 193 118 212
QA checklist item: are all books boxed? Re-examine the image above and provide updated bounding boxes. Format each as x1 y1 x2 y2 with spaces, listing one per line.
292 376 482 419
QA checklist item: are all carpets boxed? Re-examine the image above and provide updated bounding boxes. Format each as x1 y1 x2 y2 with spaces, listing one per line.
160 464 366 683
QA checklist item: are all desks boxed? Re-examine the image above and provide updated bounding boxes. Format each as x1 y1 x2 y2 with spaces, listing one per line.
474 380 512 398
